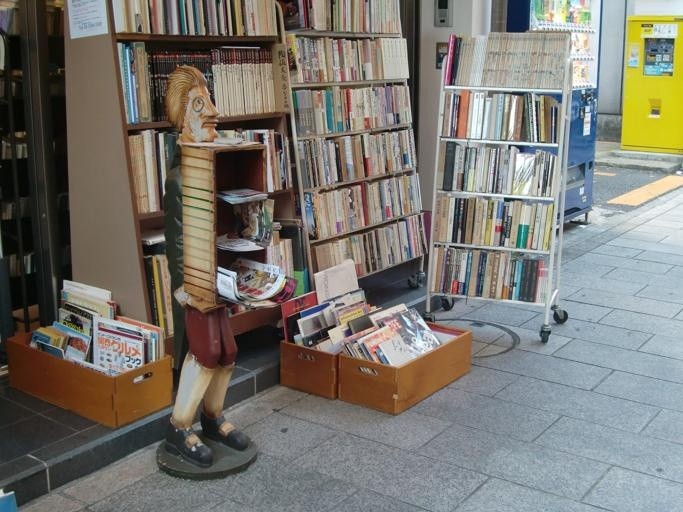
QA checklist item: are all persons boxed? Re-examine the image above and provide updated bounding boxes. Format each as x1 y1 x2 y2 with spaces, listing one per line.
152 60 253 463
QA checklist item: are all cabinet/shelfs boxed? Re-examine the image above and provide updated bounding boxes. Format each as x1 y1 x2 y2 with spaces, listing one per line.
59 0 316 367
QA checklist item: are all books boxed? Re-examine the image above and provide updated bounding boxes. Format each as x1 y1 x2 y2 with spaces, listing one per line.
109 0 428 313
29 279 165 383
430 32 569 302
0 486 20 512
1 5 65 277
280 260 440 374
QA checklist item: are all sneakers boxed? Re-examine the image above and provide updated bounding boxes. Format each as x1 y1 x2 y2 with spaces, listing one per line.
164 417 215 469
202 408 251 451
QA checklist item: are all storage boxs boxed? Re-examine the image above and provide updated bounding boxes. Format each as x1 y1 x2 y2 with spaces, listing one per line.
277 321 472 418
4 331 173 429
